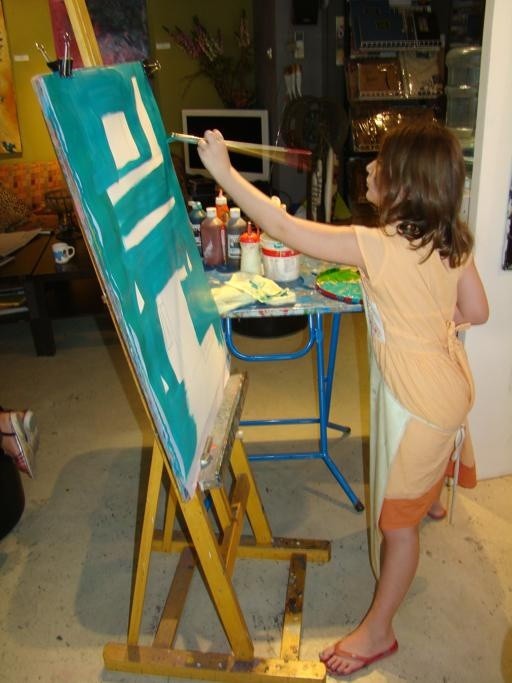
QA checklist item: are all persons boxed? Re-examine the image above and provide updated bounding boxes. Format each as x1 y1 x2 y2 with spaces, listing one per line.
0 410 40 479
198 123 489 674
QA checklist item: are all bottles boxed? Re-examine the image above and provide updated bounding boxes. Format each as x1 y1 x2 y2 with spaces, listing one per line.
190 189 267 276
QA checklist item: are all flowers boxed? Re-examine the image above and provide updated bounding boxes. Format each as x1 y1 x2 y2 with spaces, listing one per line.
160 4 273 104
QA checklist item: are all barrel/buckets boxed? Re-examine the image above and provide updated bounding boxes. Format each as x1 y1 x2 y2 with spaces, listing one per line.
260 233 301 281
445 47 481 148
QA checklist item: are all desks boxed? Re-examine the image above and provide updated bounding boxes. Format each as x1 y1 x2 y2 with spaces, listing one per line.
1 226 53 354
203 249 369 512
33 228 98 357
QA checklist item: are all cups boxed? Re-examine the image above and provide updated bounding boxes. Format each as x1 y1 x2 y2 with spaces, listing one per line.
51 242 74 262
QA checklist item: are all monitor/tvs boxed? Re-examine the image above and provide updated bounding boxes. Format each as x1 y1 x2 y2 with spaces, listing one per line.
181 108 272 183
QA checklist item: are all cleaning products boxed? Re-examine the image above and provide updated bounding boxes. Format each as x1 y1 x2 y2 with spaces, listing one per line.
190 196 260 273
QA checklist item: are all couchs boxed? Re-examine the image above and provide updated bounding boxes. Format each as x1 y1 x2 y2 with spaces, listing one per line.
0 157 81 231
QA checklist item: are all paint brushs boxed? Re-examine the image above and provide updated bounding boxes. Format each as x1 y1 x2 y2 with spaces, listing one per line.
170 128 314 173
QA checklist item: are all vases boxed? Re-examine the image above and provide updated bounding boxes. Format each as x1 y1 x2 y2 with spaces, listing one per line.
209 75 260 106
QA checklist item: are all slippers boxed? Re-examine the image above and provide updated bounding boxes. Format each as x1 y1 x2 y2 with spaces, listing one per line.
317 630 400 677
424 500 449 523
